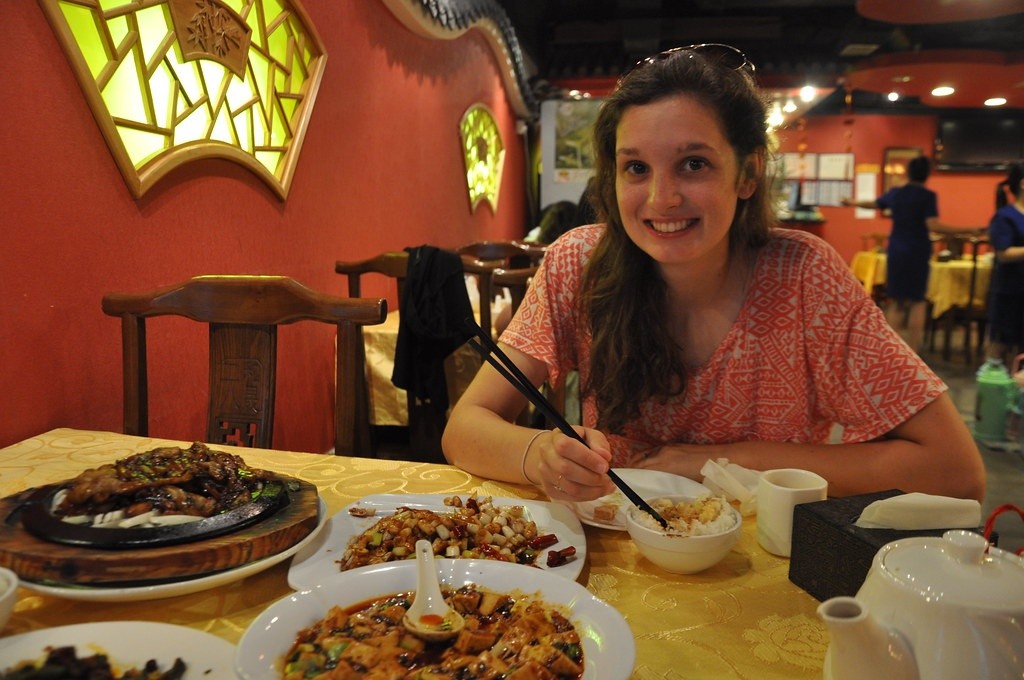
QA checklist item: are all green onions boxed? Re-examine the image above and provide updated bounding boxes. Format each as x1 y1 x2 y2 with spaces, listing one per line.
368 502 536 563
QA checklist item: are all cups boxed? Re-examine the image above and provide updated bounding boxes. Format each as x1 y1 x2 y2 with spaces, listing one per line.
757 468 829 557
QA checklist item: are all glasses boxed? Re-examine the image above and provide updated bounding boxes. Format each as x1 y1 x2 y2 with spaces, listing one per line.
611 42 754 89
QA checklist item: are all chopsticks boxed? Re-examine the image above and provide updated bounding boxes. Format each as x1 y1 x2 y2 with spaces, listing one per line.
464 319 669 527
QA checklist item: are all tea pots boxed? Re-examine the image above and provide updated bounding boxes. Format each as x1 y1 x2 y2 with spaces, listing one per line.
816 503 1024 680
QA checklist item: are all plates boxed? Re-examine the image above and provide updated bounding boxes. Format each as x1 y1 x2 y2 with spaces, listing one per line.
550 468 713 530
235 557 635 679
286 492 586 591
19 481 285 549
0 621 238 680
18 496 327 601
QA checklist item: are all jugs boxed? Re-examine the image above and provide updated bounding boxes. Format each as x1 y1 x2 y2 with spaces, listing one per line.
972 352 1024 441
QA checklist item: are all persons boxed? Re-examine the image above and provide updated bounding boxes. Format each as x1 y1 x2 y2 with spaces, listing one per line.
441 42 987 507
871 153 938 360
493 175 599 427
985 163 1023 372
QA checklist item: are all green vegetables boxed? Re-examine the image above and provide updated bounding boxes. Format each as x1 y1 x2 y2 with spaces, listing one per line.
127 455 261 514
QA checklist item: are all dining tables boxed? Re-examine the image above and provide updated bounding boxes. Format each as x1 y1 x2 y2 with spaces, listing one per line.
0 425 837 679
334 299 542 430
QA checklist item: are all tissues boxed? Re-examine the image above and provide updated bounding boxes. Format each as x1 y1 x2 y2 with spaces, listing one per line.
788 486 1000 604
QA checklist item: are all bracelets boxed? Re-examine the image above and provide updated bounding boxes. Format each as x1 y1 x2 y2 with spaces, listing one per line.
521 431 557 486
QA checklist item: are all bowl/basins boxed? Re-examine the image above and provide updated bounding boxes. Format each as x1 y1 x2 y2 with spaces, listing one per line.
624 496 742 574
1 567 19 633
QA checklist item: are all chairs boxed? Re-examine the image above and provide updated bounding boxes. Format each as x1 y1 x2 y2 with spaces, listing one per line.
100 239 990 470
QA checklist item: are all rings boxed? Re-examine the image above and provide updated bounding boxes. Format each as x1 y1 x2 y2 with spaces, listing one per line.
555 476 562 495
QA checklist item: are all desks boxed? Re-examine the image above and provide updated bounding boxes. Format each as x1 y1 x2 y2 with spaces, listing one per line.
848 250 992 347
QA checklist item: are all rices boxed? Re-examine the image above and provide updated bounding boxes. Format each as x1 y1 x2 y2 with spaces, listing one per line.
629 493 738 536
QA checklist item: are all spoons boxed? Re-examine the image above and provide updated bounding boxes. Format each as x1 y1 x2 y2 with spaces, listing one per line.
403 540 465 640
